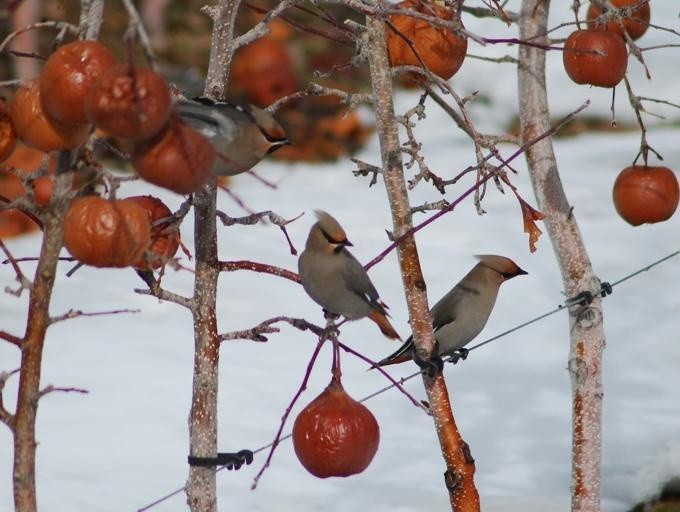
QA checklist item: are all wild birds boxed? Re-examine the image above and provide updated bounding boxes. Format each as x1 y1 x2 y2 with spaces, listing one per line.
86 95 296 181
365 253 529 379
297 209 408 346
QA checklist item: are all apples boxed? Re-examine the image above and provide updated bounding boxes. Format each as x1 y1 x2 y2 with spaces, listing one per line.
291 385 380 479
613 165 680 226
587 0 651 43
385 0 468 81
563 30 628 89
0 42 211 270
